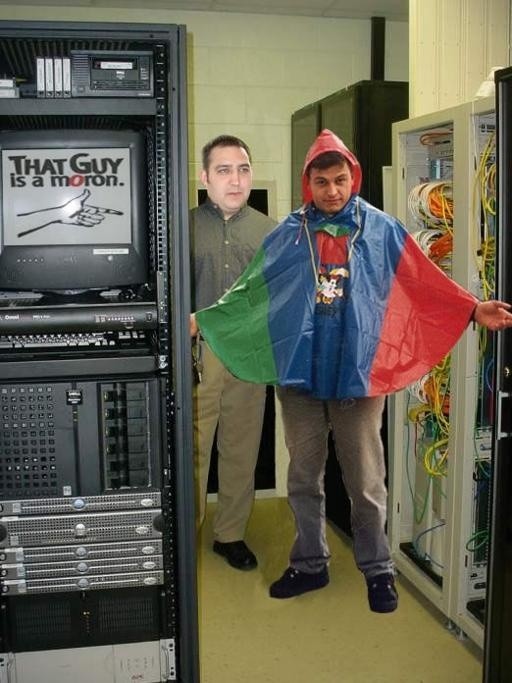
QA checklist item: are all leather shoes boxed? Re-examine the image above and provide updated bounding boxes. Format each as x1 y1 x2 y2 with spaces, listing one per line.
366 573 400 613
212 539 258 571
269 567 329 599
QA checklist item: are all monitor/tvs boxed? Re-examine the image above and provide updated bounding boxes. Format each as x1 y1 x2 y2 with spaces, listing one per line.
0 129 149 305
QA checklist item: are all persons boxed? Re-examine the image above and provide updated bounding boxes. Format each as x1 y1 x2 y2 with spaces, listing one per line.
189 120 510 616
186 133 280 572
